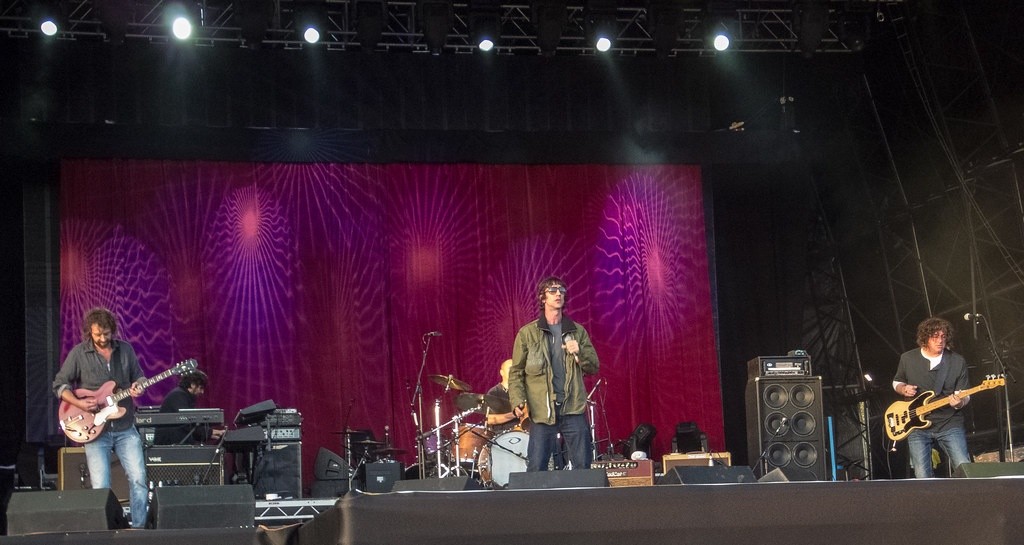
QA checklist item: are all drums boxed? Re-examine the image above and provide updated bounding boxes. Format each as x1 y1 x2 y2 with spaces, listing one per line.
452 422 495 465
423 434 448 460
478 429 530 486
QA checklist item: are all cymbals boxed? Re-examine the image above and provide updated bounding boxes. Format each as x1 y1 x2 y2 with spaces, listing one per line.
329 430 367 434
372 447 409 454
351 440 386 445
428 374 472 391
455 392 511 413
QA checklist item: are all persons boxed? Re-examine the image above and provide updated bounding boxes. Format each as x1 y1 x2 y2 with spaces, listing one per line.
485 359 520 436
508 277 600 472
153 369 237 485
52 308 149 529
893 318 972 478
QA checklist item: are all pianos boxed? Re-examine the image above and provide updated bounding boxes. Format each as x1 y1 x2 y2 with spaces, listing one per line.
217 399 277 484
134 408 224 443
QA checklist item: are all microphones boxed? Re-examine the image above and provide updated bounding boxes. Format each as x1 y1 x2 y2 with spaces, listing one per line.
964 313 981 320
565 336 580 365
587 379 601 400
79 463 85 483
428 331 443 336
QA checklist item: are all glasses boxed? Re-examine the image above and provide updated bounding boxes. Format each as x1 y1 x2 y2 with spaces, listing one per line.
544 286 566 295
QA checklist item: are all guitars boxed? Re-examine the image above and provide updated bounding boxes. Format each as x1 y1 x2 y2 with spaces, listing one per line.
58 358 199 443
884 373 1006 442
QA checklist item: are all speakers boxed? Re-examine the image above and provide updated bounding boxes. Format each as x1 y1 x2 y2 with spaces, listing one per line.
951 462 1024 478
144 443 224 499
362 462 401 494
757 466 818 482
57 447 132 501
745 376 827 482
657 466 757 485
663 452 731 475
391 475 483 492
509 467 610 489
147 485 256 531
591 459 655 486
7 488 130 536
313 449 358 480
253 441 302 499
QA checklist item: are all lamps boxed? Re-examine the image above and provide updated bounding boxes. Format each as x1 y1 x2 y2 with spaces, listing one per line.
601 424 656 459
672 421 708 453
